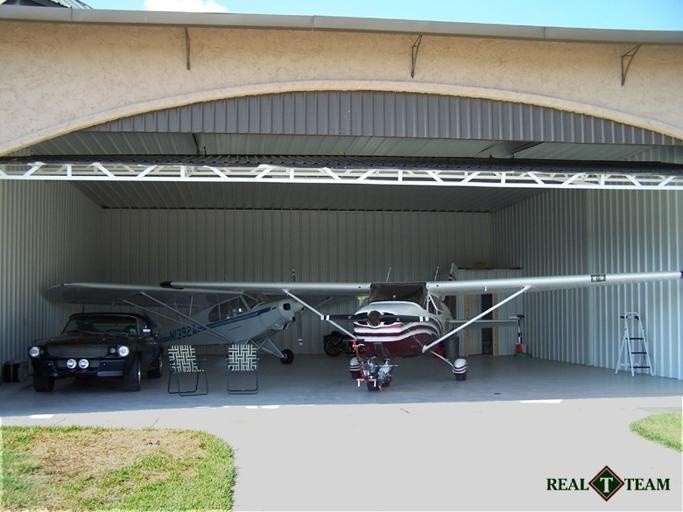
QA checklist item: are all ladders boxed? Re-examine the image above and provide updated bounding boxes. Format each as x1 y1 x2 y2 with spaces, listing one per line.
615 312 653 377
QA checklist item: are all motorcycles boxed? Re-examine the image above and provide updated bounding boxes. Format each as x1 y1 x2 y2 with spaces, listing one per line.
320 327 364 358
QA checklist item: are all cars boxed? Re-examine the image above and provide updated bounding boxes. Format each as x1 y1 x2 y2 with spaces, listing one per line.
26 310 162 391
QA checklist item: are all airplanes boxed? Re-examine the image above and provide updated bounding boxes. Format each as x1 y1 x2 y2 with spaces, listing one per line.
160 270 680 393
49 275 372 368
